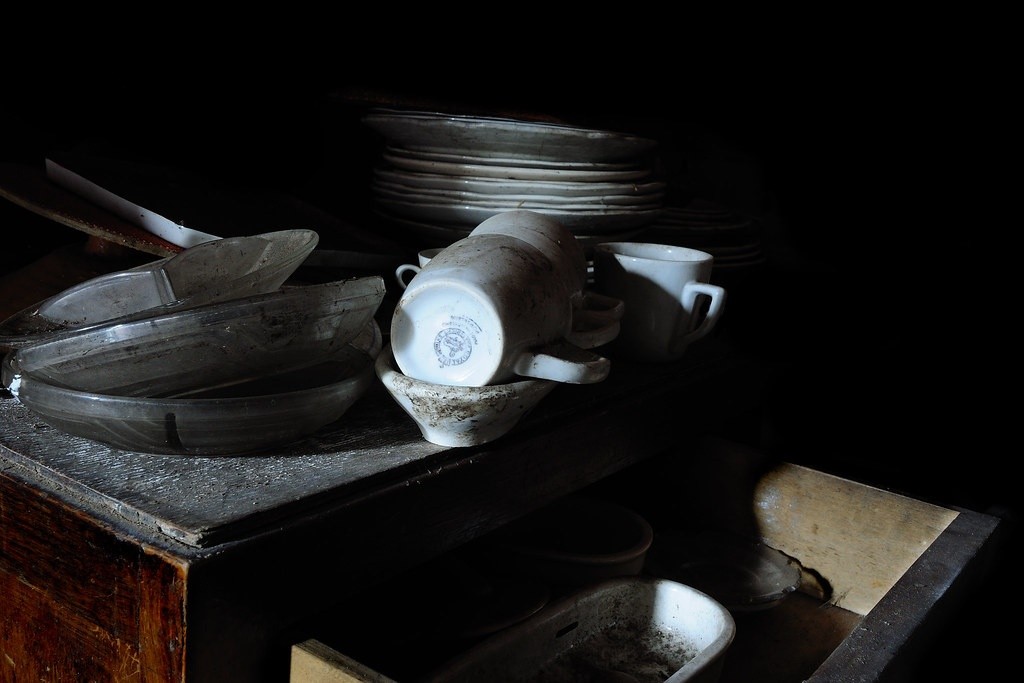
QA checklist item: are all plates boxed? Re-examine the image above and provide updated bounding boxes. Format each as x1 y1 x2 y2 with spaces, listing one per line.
0 230 319 347
2 349 374 454
17 276 387 400
358 95 777 266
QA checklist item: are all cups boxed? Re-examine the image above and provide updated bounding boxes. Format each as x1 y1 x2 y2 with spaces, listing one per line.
389 212 726 387
552 507 654 579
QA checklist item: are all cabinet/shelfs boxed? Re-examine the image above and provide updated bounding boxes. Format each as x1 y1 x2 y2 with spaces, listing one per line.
0 334 1018 683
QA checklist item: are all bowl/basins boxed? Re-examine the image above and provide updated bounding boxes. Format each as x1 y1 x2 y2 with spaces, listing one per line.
375 342 558 447
